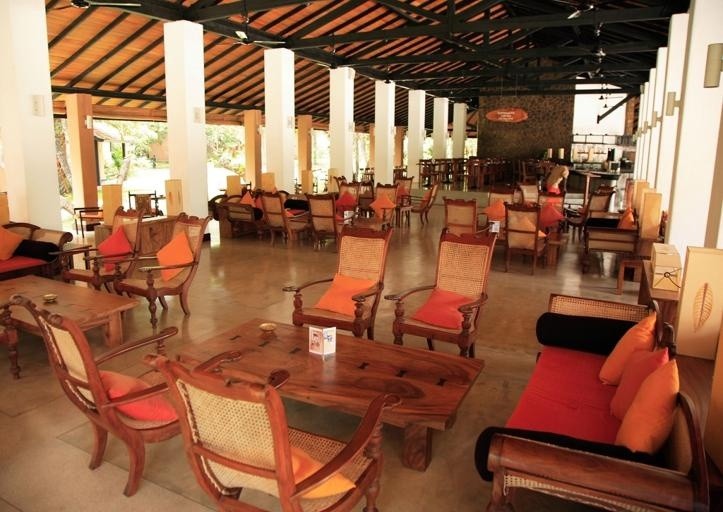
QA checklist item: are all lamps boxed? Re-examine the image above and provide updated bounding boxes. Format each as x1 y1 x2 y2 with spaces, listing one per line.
84 114 95 129
665 91 680 117
703 42 723 90
256 125 264 136
651 109 662 129
643 121 652 134
308 126 315 138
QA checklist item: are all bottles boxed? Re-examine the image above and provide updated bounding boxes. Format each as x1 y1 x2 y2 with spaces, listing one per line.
312 177 319 193
537 180 543 207
359 168 375 186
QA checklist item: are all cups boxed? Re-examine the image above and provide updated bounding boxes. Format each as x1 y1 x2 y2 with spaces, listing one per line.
260 322 277 335
44 294 59 303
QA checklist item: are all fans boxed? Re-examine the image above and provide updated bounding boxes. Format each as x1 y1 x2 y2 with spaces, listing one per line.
372 77 411 89
317 56 355 71
214 28 285 51
49 0 139 10
